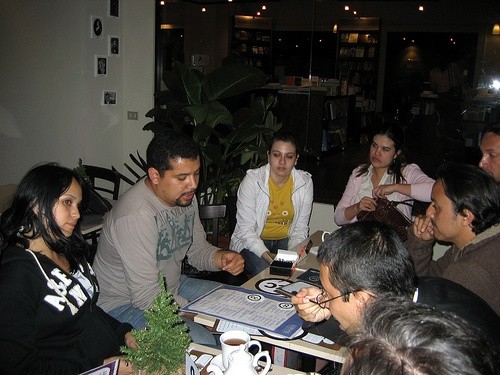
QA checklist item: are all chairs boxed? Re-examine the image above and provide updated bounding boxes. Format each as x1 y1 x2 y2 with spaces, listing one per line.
200 203 227 246
81 164 120 247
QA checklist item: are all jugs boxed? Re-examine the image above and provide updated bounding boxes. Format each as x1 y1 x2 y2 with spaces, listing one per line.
206 344 272 375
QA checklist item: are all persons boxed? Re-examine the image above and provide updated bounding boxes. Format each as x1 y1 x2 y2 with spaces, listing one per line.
0 161 143 375
406 164 500 316
339 295 500 375
90 131 246 348
228 130 314 274
429 51 465 96
333 126 436 227
476 119 500 183
288 219 500 356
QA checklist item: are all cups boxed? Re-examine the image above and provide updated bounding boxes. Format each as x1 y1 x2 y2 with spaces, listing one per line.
219 330 261 369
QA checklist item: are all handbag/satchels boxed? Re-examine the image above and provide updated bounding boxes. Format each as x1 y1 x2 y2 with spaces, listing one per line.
357 197 421 233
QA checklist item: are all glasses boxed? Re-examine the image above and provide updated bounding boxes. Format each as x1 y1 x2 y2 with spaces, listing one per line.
316 287 362 308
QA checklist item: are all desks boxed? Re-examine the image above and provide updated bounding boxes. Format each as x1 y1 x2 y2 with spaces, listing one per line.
186 230 349 365
187 341 306 375
78 195 120 235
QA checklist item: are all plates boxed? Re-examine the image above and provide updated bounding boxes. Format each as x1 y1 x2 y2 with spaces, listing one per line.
210 352 253 372
